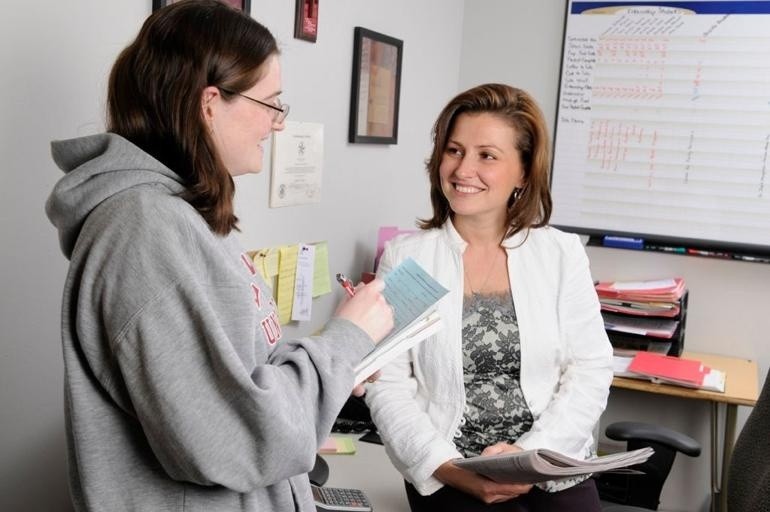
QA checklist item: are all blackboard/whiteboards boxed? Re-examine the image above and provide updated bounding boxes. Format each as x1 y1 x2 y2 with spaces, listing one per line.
540 0 770 263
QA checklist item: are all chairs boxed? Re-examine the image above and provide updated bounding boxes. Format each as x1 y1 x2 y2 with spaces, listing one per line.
599 367 770 512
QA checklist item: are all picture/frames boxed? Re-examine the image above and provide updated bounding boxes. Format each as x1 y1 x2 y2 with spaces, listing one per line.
292 0 321 43
349 25 405 145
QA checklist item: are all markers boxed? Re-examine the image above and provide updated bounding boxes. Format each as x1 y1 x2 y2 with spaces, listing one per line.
663 247 770 262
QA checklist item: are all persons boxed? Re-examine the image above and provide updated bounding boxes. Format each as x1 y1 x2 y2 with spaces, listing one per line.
45 0 396 512
360 83 613 512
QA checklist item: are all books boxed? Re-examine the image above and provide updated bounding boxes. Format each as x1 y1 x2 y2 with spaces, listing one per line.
451 446 655 483
352 258 451 391
595 278 726 393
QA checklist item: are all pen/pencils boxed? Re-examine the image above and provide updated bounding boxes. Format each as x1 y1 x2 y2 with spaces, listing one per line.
336 273 355 297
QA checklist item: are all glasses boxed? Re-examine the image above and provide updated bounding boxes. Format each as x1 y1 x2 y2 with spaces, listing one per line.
214 83 289 125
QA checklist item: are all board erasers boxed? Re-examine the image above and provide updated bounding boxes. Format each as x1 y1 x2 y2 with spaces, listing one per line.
603 235 645 249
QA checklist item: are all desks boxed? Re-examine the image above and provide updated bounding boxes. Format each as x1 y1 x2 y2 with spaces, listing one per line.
611 348 759 512
